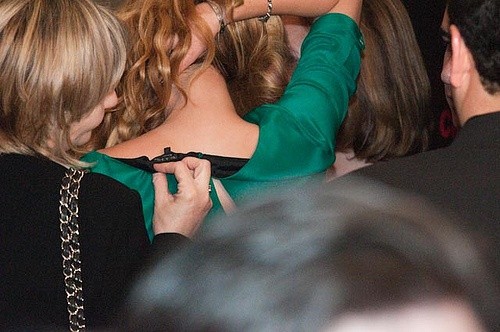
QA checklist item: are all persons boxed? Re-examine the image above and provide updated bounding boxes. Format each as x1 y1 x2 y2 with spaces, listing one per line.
285 0 434 178
325 0 499 331
78 0 367 243
439 0 462 130
0 0 212 331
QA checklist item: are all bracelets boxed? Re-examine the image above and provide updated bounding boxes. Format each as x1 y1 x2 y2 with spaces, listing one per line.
258 2 272 21
206 0 226 31
